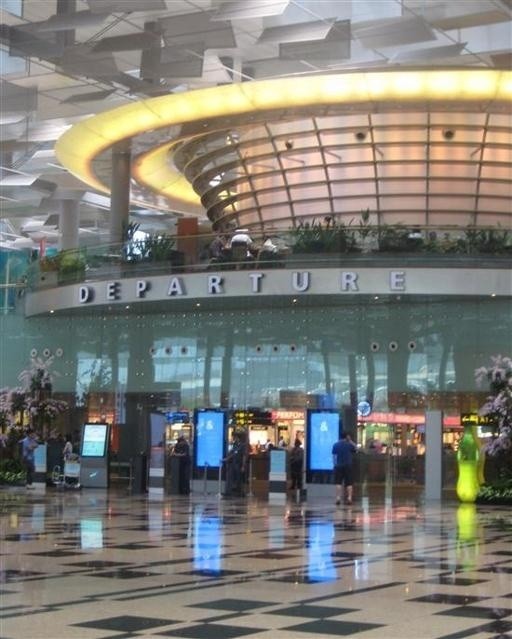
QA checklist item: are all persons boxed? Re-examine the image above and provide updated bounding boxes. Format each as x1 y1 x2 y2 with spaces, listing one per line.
255 235 273 257
23 429 39 490
62 434 73 460
255 435 285 451
224 429 249 496
229 234 252 257
211 234 225 257
330 431 357 505
408 225 422 238
331 503 355 582
289 439 304 489
285 489 305 540
19 490 36 554
169 438 191 494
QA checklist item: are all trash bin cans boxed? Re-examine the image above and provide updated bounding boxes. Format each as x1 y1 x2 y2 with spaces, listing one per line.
170 251 185 275
